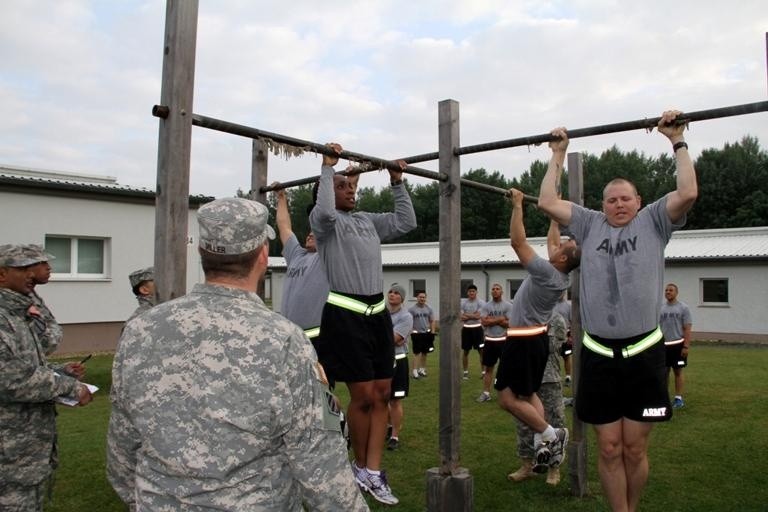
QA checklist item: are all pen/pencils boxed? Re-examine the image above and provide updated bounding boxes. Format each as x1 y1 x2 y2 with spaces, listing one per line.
69 354 91 373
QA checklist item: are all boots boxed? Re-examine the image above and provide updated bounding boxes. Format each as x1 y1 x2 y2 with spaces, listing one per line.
507 458 537 483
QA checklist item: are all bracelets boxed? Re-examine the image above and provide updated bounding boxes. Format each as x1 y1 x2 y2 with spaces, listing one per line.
673 141 688 152
391 180 403 186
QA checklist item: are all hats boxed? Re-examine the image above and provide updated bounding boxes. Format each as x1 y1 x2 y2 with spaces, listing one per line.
129 266 156 288
196 197 277 255
0 241 56 269
388 285 405 303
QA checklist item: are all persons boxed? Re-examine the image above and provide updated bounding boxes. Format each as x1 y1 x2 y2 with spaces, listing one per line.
537 110 699 511
384 281 512 451
503 187 570 467
0 241 93 512
507 312 568 485
21 243 67 357
270 180 352 450
119 266 155 337
105 197 373 511
307 142 420 506
658 283 692 409
552 288 572 387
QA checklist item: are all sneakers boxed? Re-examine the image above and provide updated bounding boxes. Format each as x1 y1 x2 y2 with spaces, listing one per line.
532 427 569 486
564 375 572 387
411 367 428 378
476 391 491 402
463 371 469 380
672 398 684 409
350 458 400 506
384 425 398 450
